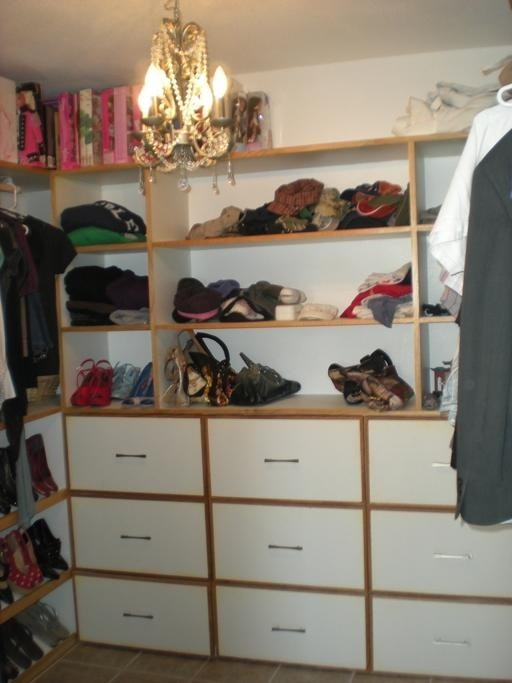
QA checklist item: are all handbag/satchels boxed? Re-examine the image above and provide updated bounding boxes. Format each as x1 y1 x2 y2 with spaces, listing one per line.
329 349 414 411
163 331 301 406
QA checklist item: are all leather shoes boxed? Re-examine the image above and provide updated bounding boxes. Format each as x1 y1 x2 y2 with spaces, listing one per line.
0 521 67 603
1 434 58 514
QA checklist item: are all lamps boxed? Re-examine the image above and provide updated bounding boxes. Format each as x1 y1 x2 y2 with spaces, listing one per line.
133 0 250 196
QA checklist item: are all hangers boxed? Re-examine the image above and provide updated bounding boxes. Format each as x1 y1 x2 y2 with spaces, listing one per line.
0 181 30 235
497 82 512 106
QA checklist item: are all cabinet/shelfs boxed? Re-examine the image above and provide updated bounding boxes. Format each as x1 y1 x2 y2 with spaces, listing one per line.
1 159 62 427
1 402 81 682
62 393 511 680
58 129 470 420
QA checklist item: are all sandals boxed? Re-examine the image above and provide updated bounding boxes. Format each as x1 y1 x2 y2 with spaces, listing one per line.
69 359 154 407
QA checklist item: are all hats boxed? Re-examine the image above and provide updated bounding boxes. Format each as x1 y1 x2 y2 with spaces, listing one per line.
297 304 338 320
268 179 324 216
355 198 396 217
340 182 380 200
173 279 282 320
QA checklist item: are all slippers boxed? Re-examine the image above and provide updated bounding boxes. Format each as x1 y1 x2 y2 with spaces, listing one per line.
1 601 71 683
